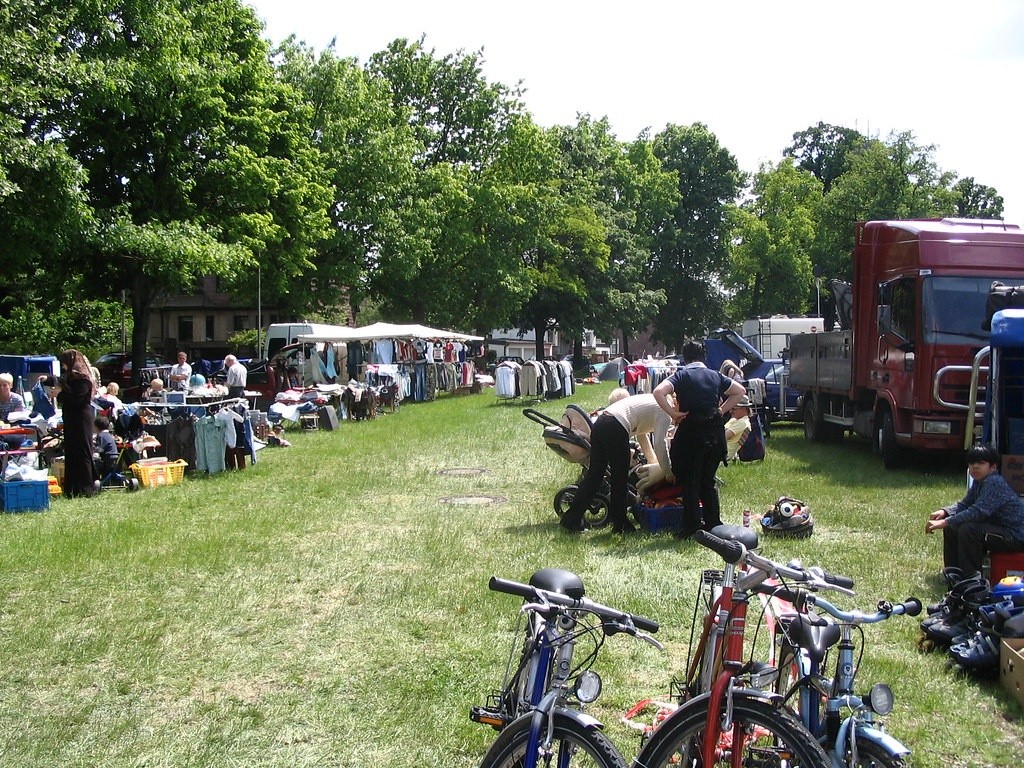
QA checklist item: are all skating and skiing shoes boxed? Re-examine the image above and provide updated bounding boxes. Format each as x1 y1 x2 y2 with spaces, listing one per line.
916 566 1024 679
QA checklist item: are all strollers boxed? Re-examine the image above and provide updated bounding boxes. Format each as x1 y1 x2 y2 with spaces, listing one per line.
522 403 651 528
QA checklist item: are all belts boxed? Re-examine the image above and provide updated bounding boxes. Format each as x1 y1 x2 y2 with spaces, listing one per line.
601 414 614 419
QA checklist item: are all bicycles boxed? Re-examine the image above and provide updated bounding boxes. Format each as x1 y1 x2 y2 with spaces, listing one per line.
751 578 923 768
470 567 667 768
628 520 857 768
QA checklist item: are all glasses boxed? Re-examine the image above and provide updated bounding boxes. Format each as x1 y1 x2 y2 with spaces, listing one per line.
730 406 738 410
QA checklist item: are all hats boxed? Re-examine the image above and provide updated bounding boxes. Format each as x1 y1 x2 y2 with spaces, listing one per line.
735 394 754 406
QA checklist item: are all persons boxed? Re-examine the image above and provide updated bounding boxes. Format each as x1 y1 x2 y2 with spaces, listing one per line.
926 446 1024 590
283 368 300 392
223 355 248 399
559 341 751 539
0 351 193 499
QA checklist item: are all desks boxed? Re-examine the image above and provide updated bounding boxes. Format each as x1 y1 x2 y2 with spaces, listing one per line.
137 398 240 419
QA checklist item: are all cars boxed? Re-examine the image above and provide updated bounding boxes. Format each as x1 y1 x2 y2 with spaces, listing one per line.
699 327 807 436
92 351 176 392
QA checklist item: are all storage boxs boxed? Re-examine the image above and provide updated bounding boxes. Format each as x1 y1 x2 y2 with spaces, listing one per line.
632 503 704 535
999 454 1024 495
129 458 188 488
999 638 1024 710
0 474 49 514
987 552 1024 586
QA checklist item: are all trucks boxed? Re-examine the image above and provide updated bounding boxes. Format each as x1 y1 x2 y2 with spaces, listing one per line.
787 215 1024 473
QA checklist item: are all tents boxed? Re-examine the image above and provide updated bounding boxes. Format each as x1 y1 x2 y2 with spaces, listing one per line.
574 356 631 381
297 322 484 411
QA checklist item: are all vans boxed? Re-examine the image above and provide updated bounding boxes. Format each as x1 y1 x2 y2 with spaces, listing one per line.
263 319 355 369
734 313 825 383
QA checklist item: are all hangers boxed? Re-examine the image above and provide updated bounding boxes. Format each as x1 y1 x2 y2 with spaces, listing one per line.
495 363 512 370
205 405 215 418
523 362 534 366
